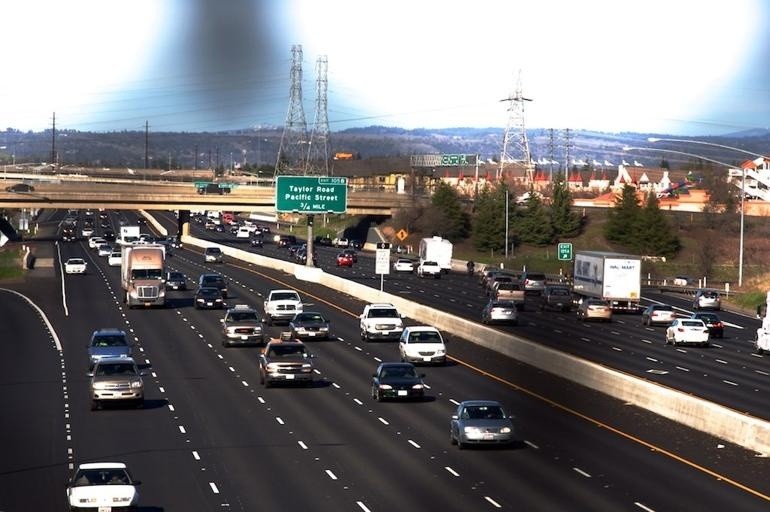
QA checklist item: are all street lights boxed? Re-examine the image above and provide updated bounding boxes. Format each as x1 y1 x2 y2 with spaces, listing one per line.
230 151 233 176
648 136 770 162
255 171 263 197
55 133 67 177
622 145 746 290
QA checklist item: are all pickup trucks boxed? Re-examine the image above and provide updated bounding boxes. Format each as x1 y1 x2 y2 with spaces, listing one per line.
196 182 231 197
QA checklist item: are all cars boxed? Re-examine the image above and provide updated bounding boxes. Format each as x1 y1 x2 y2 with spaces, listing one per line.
61 206 227 311
83 323 130 366
189 209 455 282
63 459 146 510
477 249 770 357
440 396 517 455
221 283 449 404
6 184 35 193
516 192 543 206
75 357 155 403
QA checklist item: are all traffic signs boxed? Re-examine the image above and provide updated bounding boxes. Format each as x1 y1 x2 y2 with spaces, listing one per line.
275 174 348 214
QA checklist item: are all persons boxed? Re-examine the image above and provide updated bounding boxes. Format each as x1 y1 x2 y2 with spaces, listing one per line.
109 475 124 485
467 260 475 275
488 407 499 419
